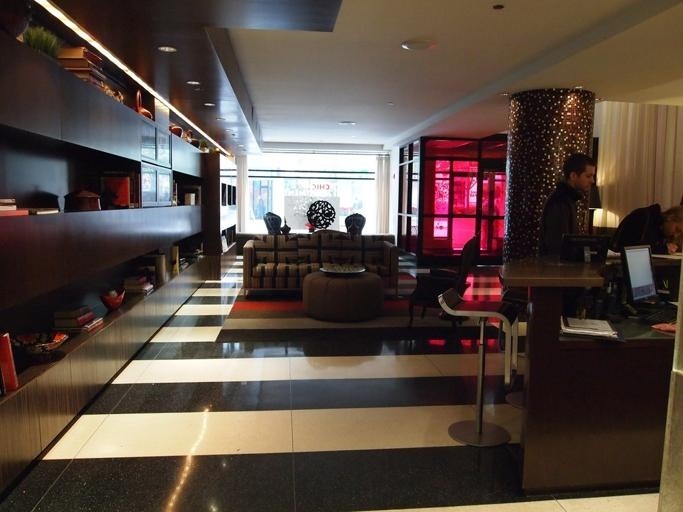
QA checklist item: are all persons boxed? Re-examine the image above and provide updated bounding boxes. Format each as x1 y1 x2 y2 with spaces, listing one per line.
538 153 595 263
608 203 683 261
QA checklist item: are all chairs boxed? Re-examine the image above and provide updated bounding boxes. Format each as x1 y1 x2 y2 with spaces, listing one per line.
407 236 481 330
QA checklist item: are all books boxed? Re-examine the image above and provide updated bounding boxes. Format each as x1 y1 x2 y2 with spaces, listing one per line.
557 315 621 341
95 170 140 209
0 196 58 219
51 246 203 331
0 332 21 392
53 42 105 89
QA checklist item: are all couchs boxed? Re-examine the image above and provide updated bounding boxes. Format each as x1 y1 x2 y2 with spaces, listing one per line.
243 232 399 296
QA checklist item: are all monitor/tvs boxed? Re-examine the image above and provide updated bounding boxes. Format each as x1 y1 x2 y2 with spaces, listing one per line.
621 244 658 315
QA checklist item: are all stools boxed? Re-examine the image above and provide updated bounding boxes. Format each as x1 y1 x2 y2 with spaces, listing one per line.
303 266 382 321
438 286 520 449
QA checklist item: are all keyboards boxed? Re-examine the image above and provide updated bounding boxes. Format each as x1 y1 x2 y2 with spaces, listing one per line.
641 305 677 327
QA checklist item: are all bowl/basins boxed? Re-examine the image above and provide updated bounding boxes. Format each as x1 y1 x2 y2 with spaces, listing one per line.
10 330 67 356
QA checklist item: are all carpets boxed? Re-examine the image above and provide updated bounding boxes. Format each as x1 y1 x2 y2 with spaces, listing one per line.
215 272 498 344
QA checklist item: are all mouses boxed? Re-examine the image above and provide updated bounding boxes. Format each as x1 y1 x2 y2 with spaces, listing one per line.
655 300 668 304
